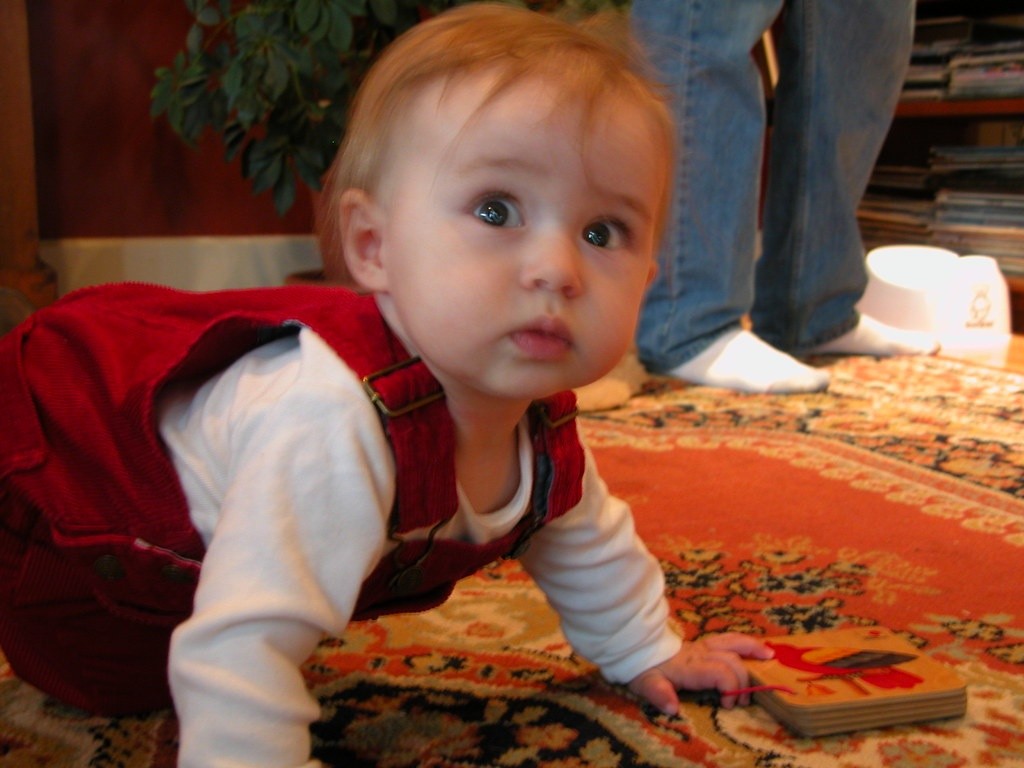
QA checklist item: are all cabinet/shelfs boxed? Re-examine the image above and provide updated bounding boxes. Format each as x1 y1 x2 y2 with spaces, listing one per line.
895 0 1024 335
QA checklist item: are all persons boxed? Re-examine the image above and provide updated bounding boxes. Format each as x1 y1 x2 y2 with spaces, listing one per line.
632 0 974 394
0 0 772 768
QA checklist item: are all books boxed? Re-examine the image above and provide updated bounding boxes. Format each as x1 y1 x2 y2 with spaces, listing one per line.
899 1 1024 100
853 140 1024 279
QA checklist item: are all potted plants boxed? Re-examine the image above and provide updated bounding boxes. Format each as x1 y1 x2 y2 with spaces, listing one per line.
150 0 456 287
